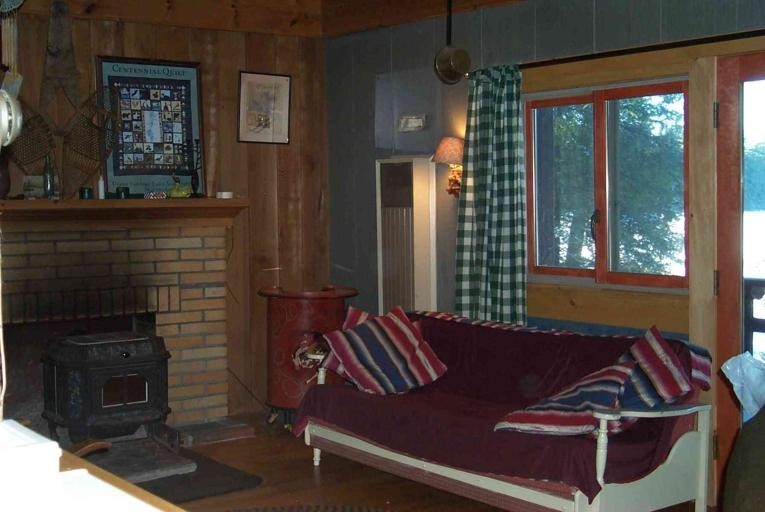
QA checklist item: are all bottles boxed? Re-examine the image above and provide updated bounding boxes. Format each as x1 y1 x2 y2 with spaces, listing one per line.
42 150 56 199
98 174 107 200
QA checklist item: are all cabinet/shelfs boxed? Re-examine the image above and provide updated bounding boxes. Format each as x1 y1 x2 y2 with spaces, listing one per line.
42 332 171 446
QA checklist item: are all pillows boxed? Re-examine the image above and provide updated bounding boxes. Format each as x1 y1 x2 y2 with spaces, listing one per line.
590 326 693 438
323 306 449 395
493 359 634 433
324 306 423 384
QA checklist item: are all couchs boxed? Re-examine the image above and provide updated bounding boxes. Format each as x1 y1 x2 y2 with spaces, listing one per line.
292 312 713 512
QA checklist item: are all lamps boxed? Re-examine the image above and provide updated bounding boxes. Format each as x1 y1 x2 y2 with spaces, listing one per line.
432 136 463 198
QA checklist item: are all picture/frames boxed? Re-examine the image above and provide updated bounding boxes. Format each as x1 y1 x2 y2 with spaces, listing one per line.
237 71 291 145
94 54 208 197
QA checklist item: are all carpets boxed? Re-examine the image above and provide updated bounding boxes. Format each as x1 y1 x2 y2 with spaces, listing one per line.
133 448 262 503
